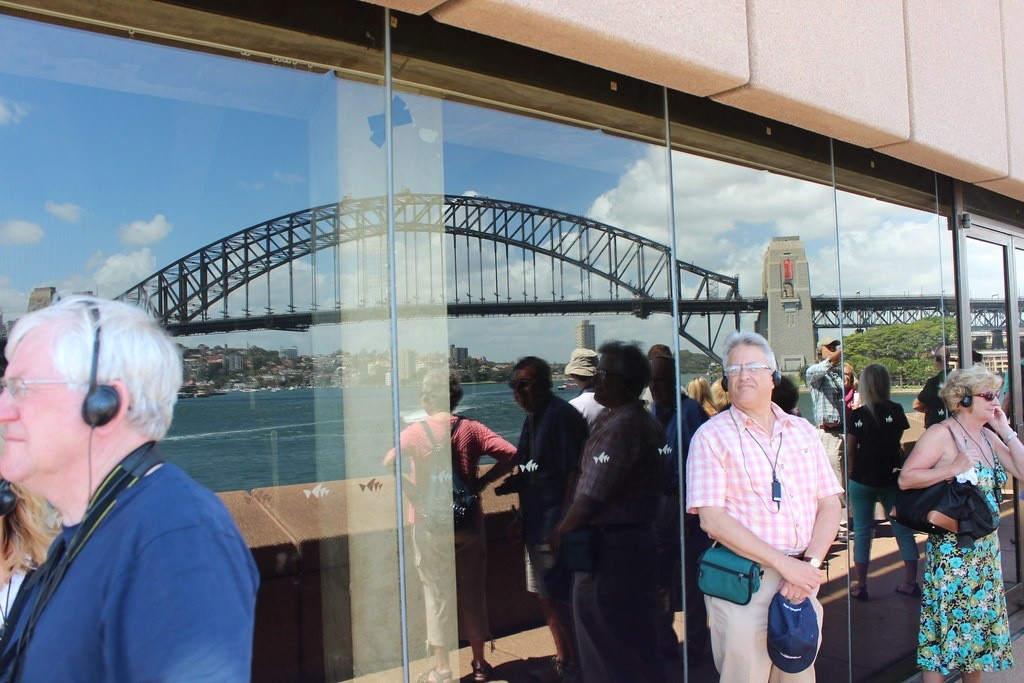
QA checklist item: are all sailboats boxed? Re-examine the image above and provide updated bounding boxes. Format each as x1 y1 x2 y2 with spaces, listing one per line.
261 378 281 392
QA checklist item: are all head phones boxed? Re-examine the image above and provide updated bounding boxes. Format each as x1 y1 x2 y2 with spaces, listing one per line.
959 386 972 407
0 479 17 517
721 357 782 392
81 299 120 428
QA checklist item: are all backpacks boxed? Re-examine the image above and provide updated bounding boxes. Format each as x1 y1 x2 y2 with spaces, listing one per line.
416 415 474 535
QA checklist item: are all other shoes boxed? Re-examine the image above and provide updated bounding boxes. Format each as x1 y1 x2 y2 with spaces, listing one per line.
895 585 921 596
850 585 868 599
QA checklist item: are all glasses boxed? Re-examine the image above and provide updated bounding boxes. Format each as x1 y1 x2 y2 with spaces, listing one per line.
725 363 769 377
508 380 539 389
975 391 999 401
0 377 78 399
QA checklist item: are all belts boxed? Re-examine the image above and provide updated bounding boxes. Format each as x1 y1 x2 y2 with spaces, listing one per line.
815 424 844 430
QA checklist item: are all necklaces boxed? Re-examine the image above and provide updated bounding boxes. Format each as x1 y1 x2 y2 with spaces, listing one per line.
952 414 996 470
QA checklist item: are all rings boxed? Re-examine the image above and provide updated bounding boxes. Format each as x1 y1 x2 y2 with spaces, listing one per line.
793 596 799 599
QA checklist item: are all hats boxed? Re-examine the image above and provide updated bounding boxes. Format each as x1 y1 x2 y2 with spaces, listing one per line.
817 337 840 348
564 348 598 376
767 592 818 673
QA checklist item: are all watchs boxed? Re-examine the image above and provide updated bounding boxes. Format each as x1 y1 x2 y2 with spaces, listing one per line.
803 556 823 570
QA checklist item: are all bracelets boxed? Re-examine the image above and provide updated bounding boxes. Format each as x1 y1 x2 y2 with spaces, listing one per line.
1004 432 1018 443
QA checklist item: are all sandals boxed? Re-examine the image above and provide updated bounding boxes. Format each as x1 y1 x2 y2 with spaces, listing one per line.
416 668 452 683
470 659 493 681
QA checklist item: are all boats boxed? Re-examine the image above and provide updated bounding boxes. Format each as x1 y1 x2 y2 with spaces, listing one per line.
558 377 577 390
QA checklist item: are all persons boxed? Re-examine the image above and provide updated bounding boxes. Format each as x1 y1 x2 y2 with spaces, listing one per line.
0 293 263 683
897 362 1024 683
685 332 845 683
382 338 1016 683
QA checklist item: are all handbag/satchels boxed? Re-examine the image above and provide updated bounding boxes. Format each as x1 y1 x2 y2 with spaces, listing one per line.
698 547 761 606
890 422 995 540
